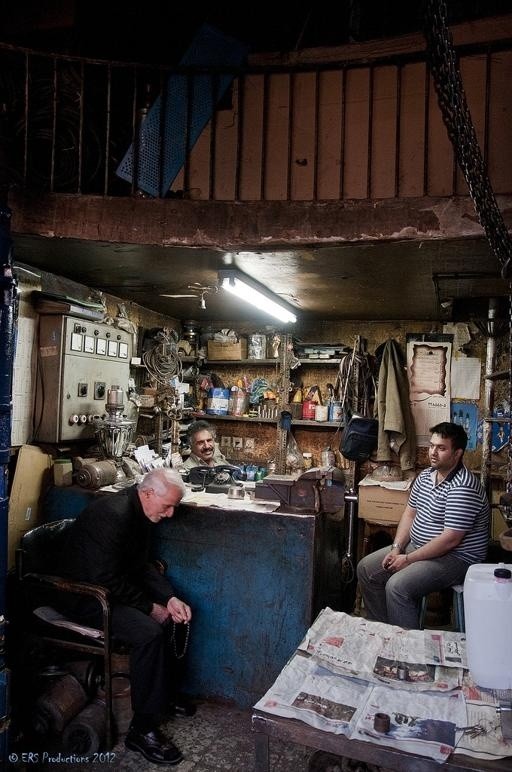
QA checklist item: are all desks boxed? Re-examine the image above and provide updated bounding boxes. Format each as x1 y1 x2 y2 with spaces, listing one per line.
249 618 512 772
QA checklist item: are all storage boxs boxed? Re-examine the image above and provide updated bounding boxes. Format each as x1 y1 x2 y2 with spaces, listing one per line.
356 472 414 522
207 338 247 361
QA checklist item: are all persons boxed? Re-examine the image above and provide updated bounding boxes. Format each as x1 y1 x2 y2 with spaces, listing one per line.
356 421 490 629
23 466 197 765
181 420 230 469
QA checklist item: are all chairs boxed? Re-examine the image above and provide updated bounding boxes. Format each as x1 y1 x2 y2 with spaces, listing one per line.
16 520 166 753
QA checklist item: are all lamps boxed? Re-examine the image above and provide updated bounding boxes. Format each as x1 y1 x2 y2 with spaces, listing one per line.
215 264 302 324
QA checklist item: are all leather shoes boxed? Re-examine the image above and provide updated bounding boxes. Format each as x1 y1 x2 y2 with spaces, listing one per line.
124 726 183 764
164 694 197 717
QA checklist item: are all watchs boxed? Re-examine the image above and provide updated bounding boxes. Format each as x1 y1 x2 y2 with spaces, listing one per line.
390 544 403 550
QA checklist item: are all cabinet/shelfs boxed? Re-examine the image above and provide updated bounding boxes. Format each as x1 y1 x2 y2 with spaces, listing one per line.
280 332 360 498
196 342 283 484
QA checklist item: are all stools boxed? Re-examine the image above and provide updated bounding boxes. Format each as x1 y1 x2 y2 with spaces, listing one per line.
356 521 401 614
417 582 465 632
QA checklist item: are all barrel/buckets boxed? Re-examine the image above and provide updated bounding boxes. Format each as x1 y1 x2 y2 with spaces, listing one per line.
463 562 512 689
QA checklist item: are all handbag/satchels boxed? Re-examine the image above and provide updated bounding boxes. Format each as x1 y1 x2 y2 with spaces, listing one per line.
338 339 379 461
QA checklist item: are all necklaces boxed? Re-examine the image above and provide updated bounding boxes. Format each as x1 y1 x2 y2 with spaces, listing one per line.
171 618 191 661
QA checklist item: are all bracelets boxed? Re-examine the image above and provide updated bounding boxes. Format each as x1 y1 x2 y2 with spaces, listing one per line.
405 554 411 565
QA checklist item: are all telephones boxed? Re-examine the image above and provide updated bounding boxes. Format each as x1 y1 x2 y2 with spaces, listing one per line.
191 464 247 494
